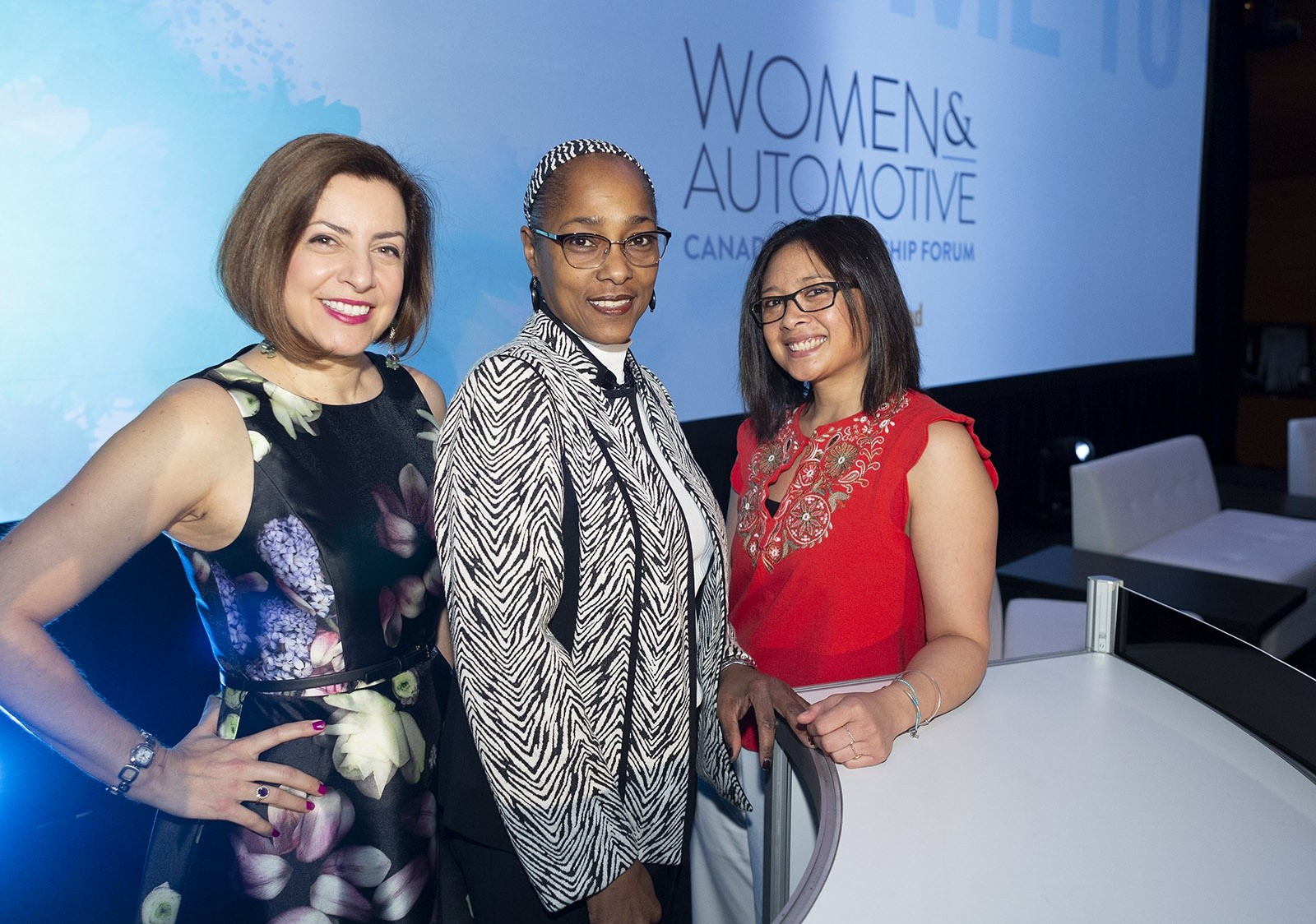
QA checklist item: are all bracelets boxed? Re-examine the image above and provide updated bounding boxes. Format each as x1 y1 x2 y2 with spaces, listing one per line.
894 669 941 738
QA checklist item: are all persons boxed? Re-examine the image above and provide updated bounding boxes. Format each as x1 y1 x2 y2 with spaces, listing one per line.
433 140 818 924
691 214 999 924
0 133 482 924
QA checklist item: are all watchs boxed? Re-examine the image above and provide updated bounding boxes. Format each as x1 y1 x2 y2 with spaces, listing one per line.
106 729 158 799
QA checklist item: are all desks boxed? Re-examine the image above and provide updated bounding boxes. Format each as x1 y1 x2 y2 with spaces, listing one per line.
992 544 1315 661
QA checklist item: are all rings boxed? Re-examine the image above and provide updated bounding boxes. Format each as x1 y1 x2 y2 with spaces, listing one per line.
850 741 862 759
843 725 863 743
256 784 270 802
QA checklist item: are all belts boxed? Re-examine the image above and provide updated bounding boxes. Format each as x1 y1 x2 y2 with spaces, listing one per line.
220 631 438 692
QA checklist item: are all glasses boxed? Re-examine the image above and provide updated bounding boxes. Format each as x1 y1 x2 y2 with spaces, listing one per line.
531 225 671 269
750 280 861 324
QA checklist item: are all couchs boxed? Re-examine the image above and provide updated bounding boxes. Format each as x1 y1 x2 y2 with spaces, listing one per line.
1072 434 1316 655
1288 417 1316 498
